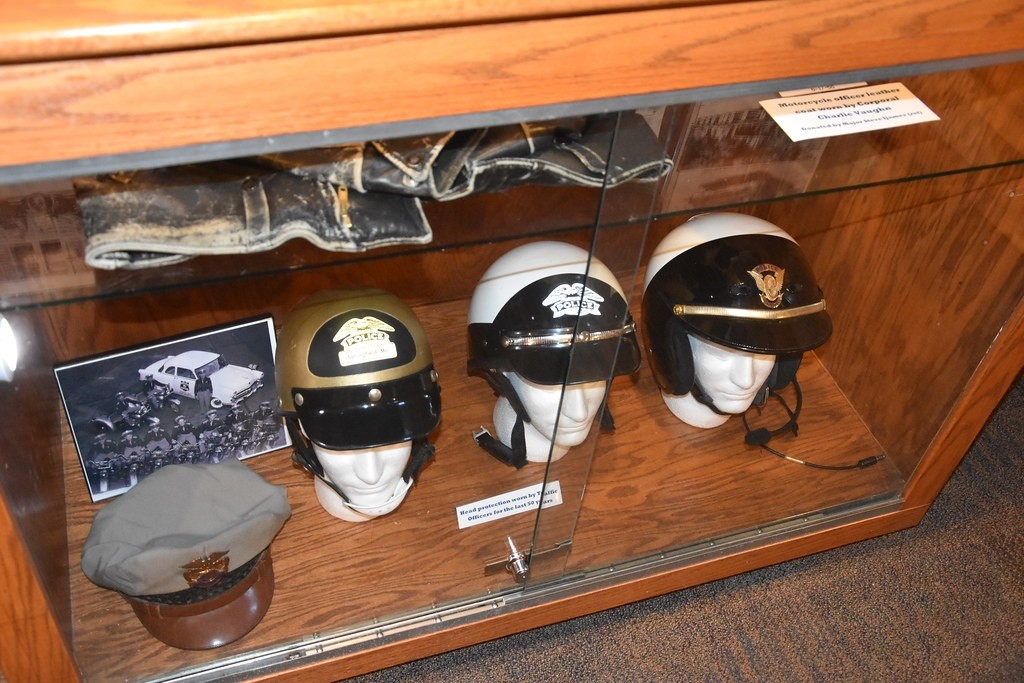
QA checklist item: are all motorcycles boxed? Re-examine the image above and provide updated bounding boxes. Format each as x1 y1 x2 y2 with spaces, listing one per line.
135 383 182 416
86 414 282 488
92 393 162 433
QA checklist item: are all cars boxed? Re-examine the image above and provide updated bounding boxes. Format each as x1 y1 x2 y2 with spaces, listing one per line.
135 350 264 408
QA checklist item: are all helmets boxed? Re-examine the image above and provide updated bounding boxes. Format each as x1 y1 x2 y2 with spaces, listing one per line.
466 240 640 385
274 285 442 451
642 211 834 396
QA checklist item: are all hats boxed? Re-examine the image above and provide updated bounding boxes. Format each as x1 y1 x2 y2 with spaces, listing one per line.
121 430 134 437
175 415 186 423
95 433 106 441
231 405 240 412
199 369 206 374
207 410 217 416
260 402 270 407
149 421 160 428
80 460 289 650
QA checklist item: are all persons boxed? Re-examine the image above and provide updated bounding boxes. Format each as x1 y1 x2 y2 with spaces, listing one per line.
143 373 169 407
115 391 142 426
85 400 280 465
466 241 641 462
194 369 214 414
273 287 442 523
640 212 835 429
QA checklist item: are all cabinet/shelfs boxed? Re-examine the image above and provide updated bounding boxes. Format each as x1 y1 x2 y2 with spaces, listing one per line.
0 0 1024 682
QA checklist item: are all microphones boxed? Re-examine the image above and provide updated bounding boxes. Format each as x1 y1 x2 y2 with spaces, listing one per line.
745 375 803 446
